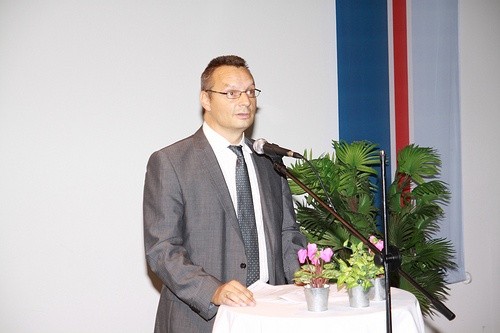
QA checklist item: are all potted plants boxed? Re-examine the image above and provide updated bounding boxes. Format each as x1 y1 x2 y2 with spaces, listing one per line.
332 242 372 308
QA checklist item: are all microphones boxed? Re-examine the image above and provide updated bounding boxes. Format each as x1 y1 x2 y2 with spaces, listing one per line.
253 139 303 160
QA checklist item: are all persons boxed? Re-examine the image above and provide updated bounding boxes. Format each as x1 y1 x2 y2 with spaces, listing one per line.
143 55 312 333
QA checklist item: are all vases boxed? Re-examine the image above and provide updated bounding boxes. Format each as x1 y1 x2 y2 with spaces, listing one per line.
368 278 386 301
304 285 329 311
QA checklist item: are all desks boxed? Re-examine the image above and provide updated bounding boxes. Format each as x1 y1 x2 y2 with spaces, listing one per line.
213 283 425 333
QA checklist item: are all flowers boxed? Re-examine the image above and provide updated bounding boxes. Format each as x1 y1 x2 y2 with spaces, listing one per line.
294 244 332 287
367 236 384 278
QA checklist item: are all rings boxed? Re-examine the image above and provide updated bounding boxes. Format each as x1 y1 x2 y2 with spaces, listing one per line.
225 291 231 297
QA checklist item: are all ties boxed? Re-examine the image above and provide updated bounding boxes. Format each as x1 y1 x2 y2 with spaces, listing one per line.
227 144 260 288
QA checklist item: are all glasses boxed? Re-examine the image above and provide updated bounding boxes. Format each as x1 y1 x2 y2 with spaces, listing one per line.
205 88 261 98
